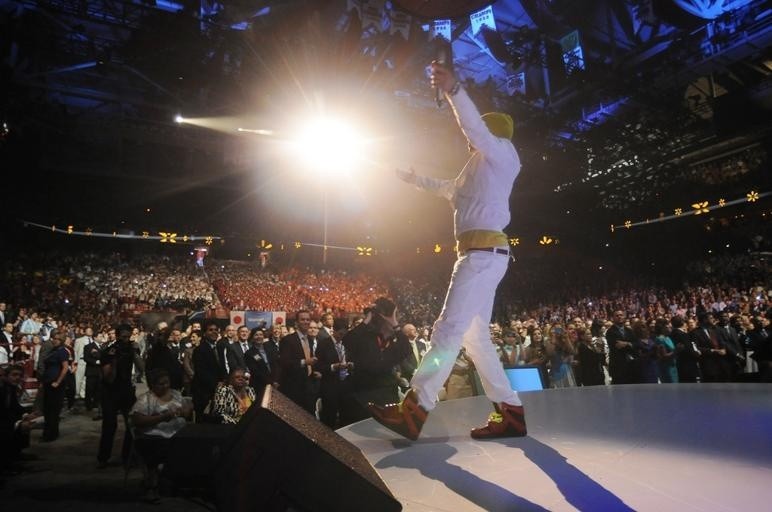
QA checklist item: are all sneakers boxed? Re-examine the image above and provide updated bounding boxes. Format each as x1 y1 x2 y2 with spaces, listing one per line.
97 457 108 467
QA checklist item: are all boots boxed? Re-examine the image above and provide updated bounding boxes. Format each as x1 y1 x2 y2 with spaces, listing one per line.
367 389 429 441
471 401 527 439
144 478 161 501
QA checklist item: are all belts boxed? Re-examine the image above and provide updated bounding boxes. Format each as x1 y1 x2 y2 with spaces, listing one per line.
469 247 511 255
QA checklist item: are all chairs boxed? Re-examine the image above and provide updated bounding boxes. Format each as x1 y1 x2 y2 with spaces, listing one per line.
121 403 148 485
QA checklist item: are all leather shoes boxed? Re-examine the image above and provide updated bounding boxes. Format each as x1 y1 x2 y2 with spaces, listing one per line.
16 451 37 460
4 464 23 475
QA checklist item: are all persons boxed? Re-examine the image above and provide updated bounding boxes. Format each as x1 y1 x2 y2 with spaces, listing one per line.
368 60 528 442
2 229 771 500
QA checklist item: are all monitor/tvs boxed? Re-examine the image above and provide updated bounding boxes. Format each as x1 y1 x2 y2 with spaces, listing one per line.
468 363 548 396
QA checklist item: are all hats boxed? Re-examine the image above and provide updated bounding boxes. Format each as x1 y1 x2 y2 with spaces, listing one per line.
480 112 514 141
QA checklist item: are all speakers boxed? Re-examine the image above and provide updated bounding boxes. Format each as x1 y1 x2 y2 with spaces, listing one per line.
203 384 402 512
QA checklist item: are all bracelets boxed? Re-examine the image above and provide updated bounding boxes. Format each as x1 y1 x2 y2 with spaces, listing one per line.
446 80 461 97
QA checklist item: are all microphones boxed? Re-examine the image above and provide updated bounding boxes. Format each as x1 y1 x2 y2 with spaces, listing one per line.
431 50 446 108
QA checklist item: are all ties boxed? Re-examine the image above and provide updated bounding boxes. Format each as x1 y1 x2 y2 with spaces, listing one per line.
302 336 312 376
243 343 247 352
